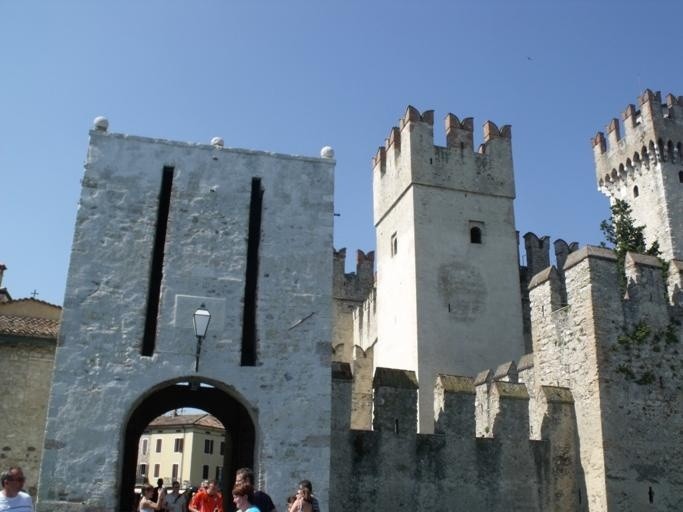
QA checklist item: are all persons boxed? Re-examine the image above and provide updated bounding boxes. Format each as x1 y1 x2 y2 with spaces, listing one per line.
233 483 261 512
235 468 277 512
286 487 312 512
297 480 321 511
137 478 226 512
0 465 36 512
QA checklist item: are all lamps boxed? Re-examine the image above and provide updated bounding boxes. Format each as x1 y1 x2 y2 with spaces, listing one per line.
191 302 211 374
